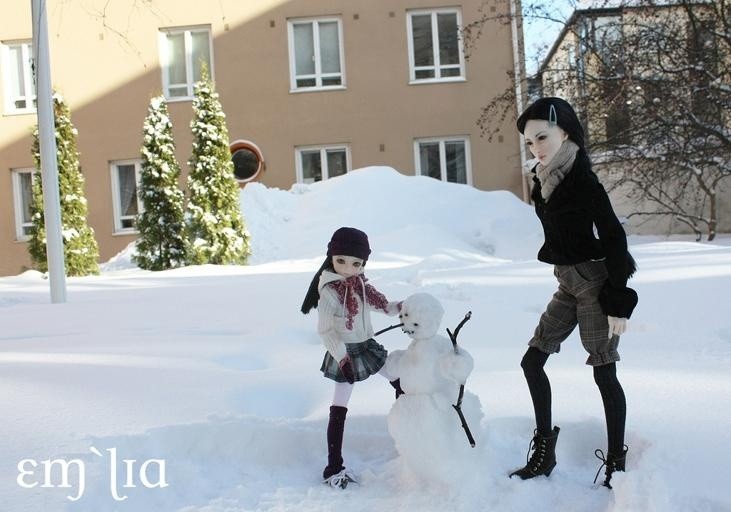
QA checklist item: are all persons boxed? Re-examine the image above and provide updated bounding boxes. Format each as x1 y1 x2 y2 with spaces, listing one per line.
299 225 404 491
508 97 642 492
